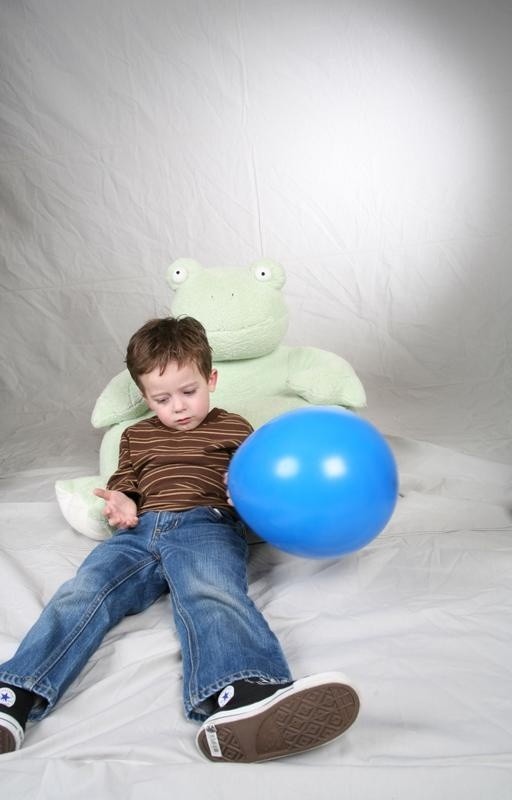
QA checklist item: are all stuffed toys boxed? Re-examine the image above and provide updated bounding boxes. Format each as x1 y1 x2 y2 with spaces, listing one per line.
53 255 368 540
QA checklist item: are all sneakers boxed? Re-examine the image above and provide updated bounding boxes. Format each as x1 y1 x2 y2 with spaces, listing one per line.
195 676 365 768
0 680 36 754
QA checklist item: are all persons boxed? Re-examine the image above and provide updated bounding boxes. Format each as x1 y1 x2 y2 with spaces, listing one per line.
0 314 362 766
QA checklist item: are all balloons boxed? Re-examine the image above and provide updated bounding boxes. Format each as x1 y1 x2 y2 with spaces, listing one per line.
225 406 399 559
